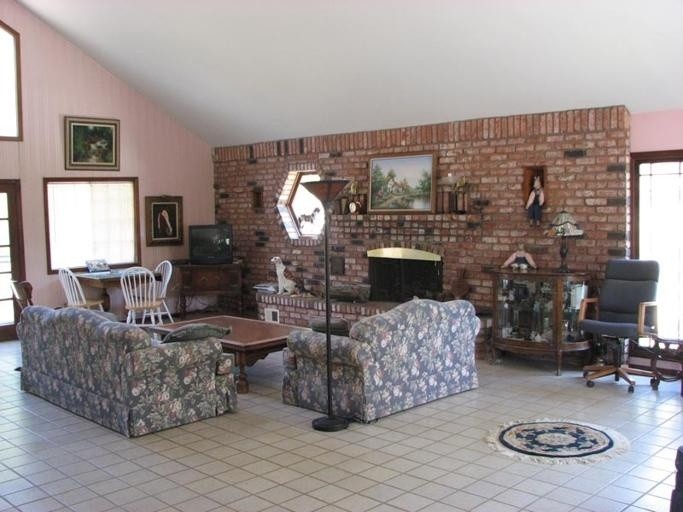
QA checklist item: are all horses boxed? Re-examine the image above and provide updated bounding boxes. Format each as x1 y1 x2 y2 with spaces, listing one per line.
156 205 174 238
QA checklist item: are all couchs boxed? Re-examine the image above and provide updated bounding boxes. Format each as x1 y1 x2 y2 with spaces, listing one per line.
281 300 480 423
16 304 237 437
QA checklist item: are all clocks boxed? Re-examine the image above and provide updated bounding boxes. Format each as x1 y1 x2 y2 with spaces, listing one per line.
348 201 362 214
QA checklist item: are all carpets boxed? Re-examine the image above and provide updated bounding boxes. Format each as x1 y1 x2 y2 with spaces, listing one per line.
487 417 629 465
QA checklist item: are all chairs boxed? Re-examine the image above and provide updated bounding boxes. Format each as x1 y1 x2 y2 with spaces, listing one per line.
577 260 660 392
9 260 176 325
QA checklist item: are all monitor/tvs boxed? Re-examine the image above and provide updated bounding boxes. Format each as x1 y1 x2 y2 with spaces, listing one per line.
188 223 234 265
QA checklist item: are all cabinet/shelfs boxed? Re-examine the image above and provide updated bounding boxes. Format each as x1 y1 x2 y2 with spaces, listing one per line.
482 267 595 376
179 263 241 320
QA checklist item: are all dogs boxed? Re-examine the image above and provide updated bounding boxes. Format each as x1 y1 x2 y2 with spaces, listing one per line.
298 207 321 229
270 256 311 296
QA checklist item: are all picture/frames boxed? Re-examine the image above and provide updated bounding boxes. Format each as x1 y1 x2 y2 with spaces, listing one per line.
64 118 121 171
366 152 437 215
145 196 183 246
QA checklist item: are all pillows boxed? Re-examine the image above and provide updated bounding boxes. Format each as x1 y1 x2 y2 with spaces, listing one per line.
311 317 348 337
162 324 230 344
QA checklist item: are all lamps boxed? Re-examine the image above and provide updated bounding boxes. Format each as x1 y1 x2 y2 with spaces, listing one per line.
547 211 583 275
300 181 350 432
